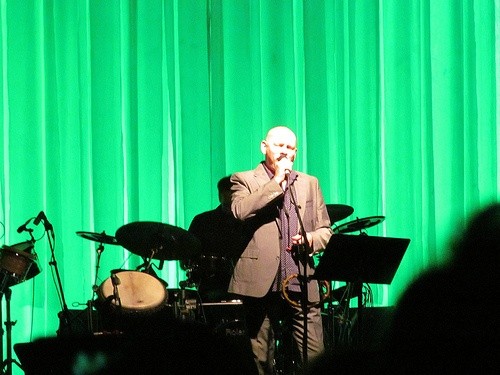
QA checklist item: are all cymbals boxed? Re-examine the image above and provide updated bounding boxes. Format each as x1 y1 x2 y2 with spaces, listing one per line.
325 203 354 224
75 230 118 246
114 221 201 262
331 215 388 234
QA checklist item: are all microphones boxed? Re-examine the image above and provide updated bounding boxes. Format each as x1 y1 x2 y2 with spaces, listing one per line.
33 211 43 225
278 155 290 175
17 218 32 233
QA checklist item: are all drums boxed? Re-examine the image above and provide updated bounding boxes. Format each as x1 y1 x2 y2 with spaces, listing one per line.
191 254 235 293
95 269 169 317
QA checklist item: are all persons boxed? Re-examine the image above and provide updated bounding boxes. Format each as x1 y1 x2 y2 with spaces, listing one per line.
178 179 253 309
4 203 500 375
228 126 332 367
